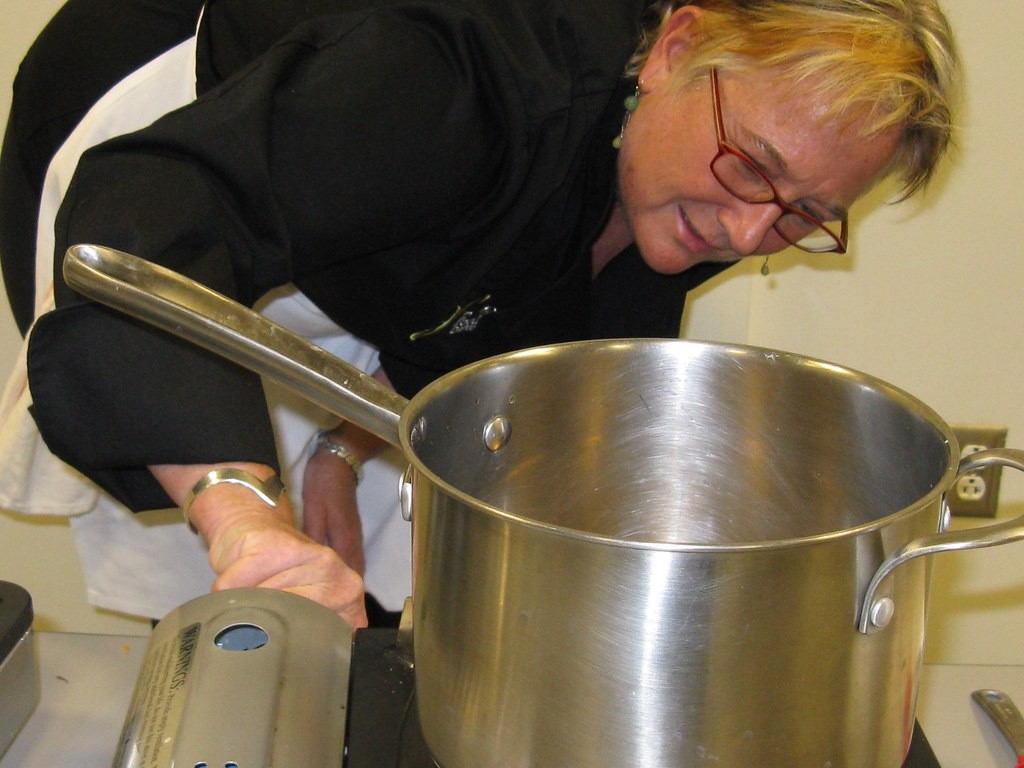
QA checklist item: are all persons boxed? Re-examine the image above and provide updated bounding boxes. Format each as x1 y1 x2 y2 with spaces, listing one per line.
2 1 968 641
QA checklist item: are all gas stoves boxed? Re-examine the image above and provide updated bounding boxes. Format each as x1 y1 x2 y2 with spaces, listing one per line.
107 586 940 768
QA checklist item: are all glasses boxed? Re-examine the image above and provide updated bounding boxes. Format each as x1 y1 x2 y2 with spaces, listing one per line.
707 66 849 253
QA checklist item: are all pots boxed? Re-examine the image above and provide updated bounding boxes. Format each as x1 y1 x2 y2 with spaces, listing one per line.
62 240 1024 768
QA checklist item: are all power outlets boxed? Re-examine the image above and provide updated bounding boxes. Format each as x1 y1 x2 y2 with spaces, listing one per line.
946 426 1008 518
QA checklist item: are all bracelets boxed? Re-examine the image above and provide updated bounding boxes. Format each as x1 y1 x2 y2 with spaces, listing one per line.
183 467 287 536
318 434 365 486
